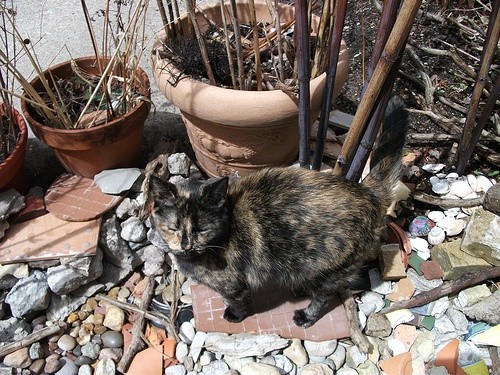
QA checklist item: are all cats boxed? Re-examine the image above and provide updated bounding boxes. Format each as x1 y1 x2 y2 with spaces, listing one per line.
148 105 410 329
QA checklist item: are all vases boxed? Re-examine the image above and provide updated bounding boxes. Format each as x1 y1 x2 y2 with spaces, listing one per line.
151 0 349 180
21 55 151 178
0 102 27 192
